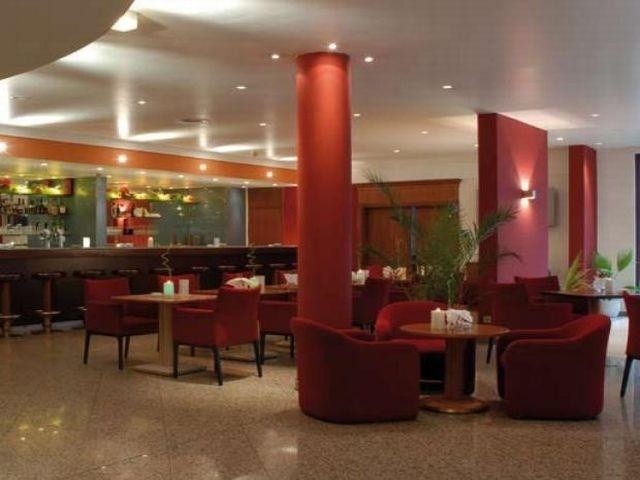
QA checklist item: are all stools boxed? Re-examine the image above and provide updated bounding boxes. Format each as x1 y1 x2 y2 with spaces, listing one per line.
30 269 67 337
0 270 24 340
71 269 104 332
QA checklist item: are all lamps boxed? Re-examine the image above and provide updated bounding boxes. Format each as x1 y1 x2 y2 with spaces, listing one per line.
517 189 537 199
109 10 138 36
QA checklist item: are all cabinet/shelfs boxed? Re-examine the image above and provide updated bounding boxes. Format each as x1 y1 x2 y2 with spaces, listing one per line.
0 189 70 237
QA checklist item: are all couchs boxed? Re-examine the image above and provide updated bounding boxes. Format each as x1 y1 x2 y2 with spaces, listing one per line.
292 317 420 424
496 314 611 421
374 299 464 356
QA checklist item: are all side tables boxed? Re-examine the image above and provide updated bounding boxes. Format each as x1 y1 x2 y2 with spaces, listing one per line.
401 323 510 414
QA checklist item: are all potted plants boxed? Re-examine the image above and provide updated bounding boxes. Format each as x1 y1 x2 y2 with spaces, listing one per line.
590 250 635 318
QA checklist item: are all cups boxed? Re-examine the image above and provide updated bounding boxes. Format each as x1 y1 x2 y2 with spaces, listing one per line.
81 236 91 248
428 309 447 331
178 278 191 299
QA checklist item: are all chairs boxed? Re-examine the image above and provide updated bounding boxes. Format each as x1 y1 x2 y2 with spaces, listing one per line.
620 290 640 398
81 268 297 385
351 266 421 328
464 274 623 364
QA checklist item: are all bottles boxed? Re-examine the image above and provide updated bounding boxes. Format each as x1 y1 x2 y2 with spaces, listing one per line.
4 194 68 231
